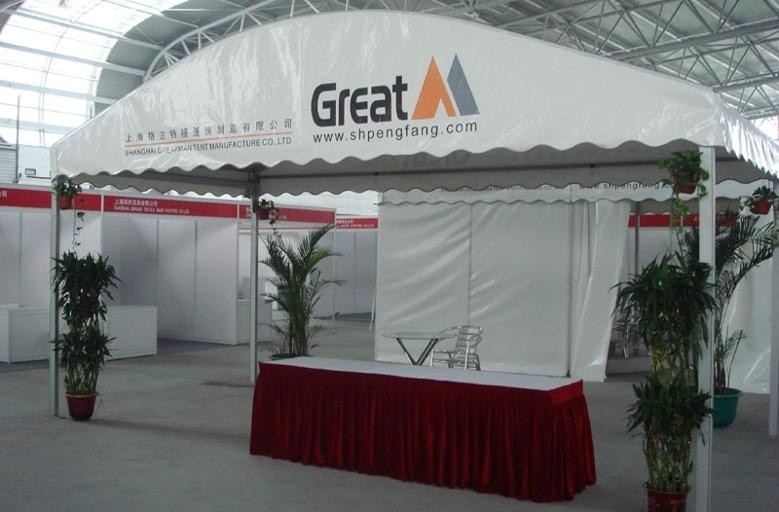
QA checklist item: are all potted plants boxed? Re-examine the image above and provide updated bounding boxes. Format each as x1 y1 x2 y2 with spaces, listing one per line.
607 248 720 512
671 214 779 427
47 248 124 422
737 185 779 215
657 148 710 233
251 196 281 239
253 221 344 363
50 173 85 247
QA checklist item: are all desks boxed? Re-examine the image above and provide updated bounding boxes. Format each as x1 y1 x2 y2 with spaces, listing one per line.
382 332 455 365
250 356 596 504
237 299 272 343
99 304 160 361
0 303 51 365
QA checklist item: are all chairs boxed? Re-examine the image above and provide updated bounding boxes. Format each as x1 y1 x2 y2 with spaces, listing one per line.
430 324 484 371
